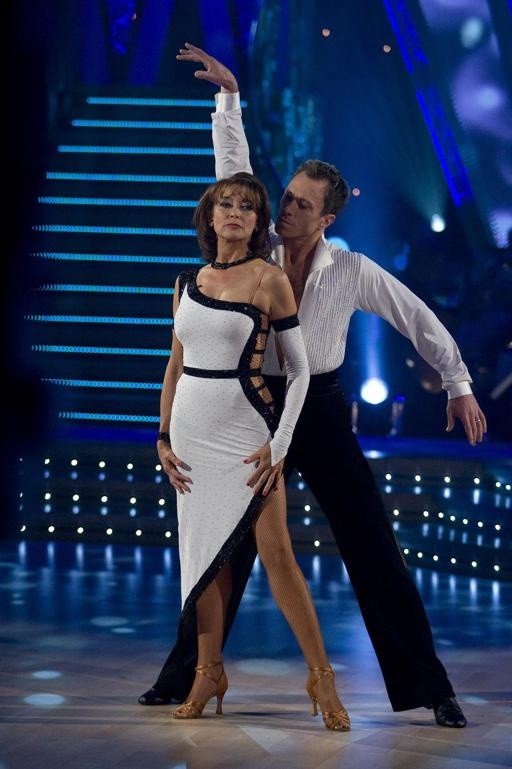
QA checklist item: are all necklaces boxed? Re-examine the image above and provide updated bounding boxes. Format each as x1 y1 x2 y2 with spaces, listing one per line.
211 253 257 269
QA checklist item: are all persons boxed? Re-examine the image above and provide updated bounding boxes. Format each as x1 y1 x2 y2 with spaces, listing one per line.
137 42 488 728
154 170 352 731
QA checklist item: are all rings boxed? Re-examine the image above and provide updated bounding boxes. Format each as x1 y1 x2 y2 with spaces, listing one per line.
477 420 480 422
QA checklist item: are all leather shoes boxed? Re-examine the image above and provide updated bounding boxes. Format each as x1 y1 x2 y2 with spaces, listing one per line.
424 696 466 728
138 685 178 704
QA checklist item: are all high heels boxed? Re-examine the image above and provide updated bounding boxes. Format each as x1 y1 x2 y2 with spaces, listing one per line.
172 661 228 720
306 665 352 731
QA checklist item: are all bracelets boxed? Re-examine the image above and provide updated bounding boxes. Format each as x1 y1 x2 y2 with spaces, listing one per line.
156 432 170 441
